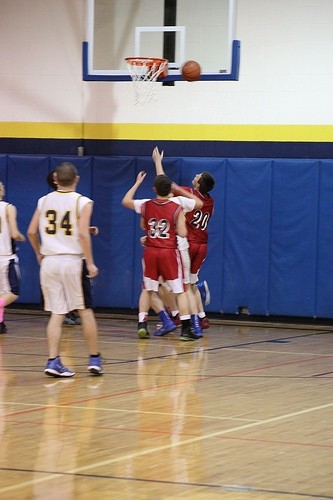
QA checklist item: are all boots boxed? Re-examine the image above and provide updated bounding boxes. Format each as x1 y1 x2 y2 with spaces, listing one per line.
155 310 177 336
179 315 199 340
191 314 203 338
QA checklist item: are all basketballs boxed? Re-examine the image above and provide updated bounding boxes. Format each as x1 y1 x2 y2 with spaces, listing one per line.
181 61 200 81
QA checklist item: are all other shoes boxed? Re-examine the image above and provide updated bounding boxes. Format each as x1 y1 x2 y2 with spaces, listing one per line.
0 321 7 334
62 316 75 327
198 316 210 329
166 306 181 325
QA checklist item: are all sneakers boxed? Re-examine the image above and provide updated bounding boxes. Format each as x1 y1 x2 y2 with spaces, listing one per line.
87 356 103 376
137 322 150 339
44 357 76 377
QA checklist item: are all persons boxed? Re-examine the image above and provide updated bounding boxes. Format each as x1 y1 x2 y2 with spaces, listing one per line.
0 181 25 334
61 227 98 326
27 163 104 377
121 146 215 340
